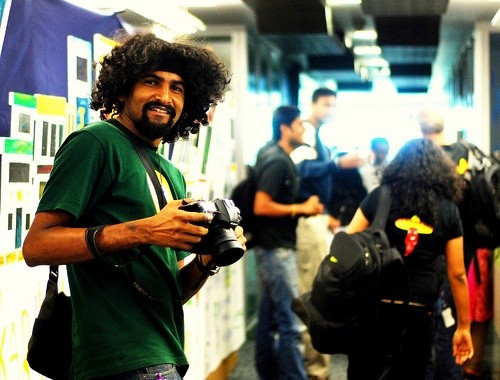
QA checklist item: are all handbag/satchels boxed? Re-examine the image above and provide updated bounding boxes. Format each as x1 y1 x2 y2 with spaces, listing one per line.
27 290 75 380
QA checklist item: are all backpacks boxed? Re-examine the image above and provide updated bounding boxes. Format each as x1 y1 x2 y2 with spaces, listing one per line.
231 177 260 250
308 227 402 355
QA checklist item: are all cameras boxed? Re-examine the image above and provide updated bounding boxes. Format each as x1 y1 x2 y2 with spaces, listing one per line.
178 198 245 267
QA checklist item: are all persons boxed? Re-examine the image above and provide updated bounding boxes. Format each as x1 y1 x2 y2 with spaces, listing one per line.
345 138 472 380
363 137 393 195
463 242 491 375
412 105 483 380
290 88 364 380
253 105 325 379
21 33 247 380
